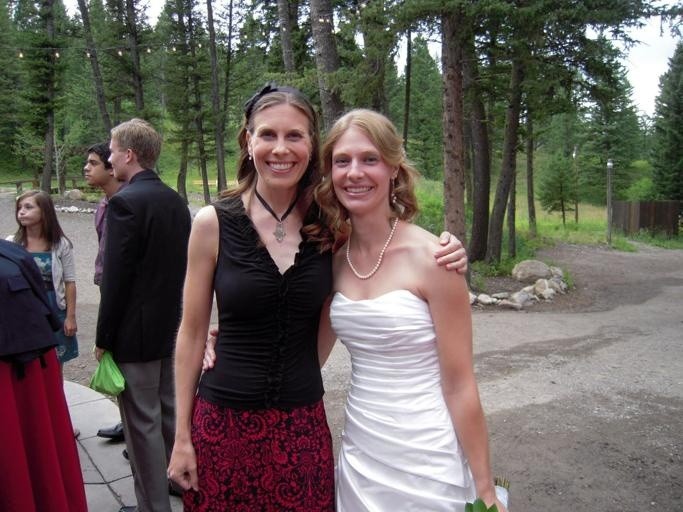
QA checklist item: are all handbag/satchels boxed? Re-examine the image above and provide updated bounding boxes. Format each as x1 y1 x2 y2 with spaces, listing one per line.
88 350 126 396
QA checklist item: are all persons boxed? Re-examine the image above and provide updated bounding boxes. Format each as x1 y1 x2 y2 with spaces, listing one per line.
165 78 471 512
0 238 89 511
200 106 509 512
94 115 192 512
82 140 131 460
5 188 81 439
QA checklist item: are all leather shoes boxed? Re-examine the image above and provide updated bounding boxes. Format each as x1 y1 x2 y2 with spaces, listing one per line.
119 506 137 512
97 423 125 438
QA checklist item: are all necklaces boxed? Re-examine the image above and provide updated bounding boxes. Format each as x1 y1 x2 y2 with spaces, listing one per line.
253 180 302 242
345 216 400 280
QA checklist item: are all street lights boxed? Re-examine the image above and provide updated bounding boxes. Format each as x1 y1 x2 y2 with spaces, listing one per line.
605 158 615 246
